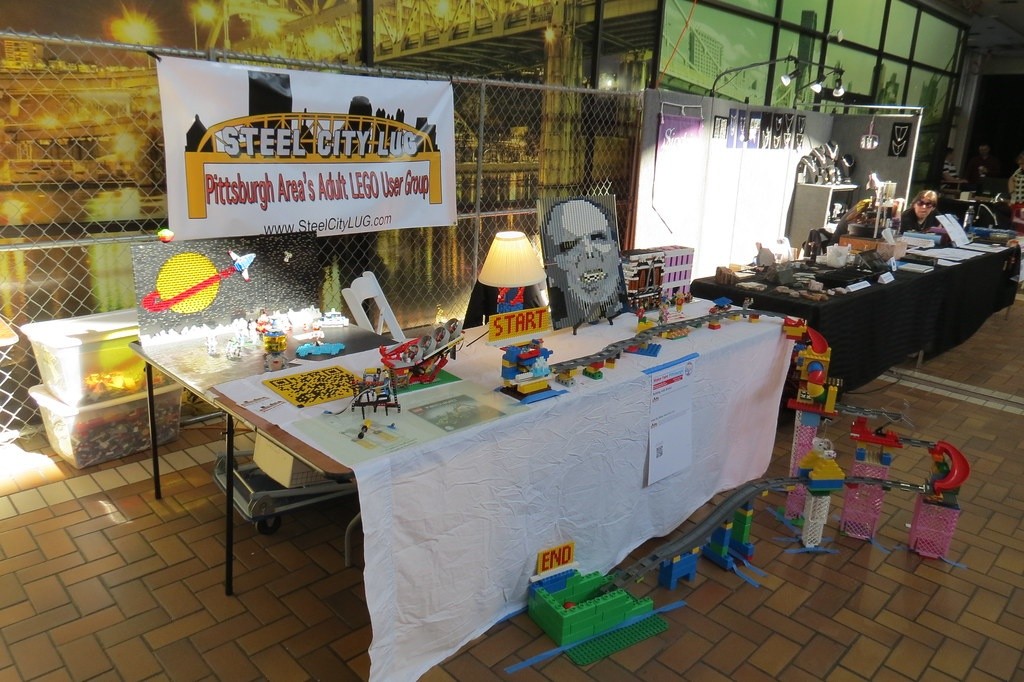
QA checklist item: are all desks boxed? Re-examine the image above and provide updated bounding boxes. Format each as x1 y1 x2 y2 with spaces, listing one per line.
690 199 1021 426
128 294 806 682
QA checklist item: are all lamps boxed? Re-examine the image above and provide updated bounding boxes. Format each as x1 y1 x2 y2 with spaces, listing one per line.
477 233 547 310
709 55 800 97
832 72 844 96
810 67 826 93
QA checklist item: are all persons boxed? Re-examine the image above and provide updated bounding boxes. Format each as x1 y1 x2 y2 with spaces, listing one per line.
900 190 942 235
939 147 960 180
963 144 1001 196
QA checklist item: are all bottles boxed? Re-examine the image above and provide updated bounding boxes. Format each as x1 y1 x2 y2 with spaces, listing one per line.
963 205 975 228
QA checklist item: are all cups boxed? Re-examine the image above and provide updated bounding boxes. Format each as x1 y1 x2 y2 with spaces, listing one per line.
826 243 848 268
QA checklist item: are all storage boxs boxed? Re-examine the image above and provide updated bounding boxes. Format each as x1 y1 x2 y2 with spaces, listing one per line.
19 308 177 408
28 383 183 470
251 434 334 489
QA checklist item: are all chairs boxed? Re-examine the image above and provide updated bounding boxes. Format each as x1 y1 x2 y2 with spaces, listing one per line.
342 271 416 343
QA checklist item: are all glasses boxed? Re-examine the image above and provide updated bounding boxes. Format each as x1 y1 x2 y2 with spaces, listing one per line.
917 201 932 208
